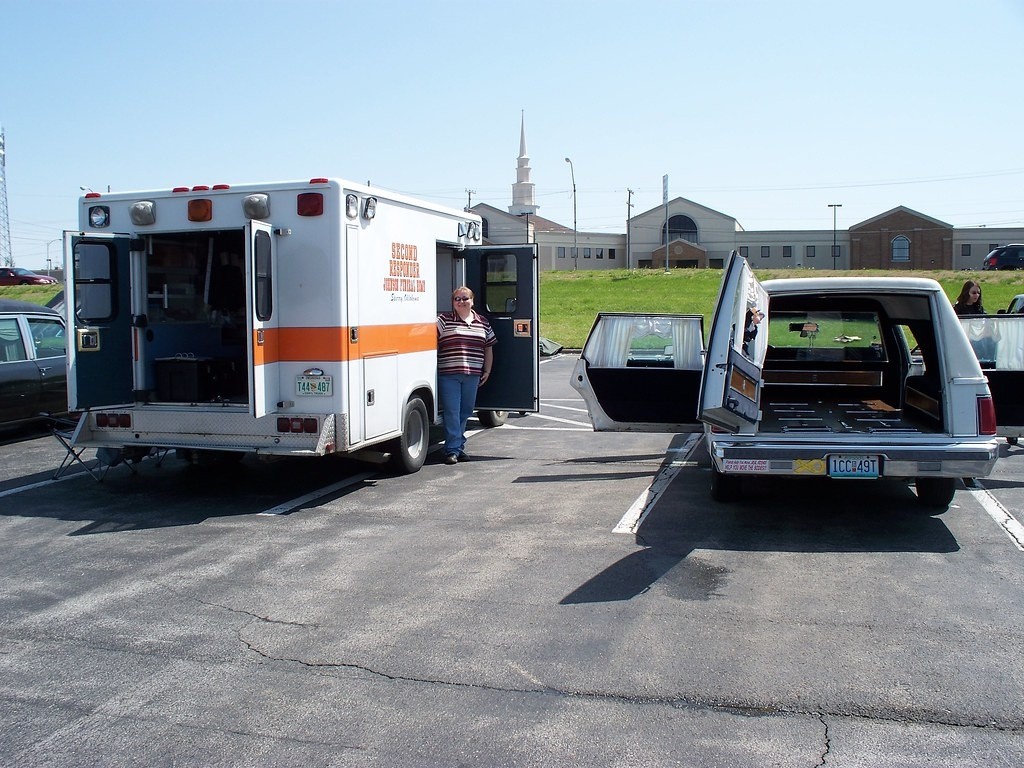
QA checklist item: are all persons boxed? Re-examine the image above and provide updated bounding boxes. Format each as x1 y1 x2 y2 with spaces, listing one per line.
951 280 996 372
437 286 498 464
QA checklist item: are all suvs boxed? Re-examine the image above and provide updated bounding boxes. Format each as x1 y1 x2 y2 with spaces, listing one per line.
569 251 1007 515
982 243 1024 271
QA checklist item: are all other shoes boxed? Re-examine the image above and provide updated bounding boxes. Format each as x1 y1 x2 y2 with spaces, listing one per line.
446 453 457 464
457 451 470 462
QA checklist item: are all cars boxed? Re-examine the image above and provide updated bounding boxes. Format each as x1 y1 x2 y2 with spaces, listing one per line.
0 298 81 439
0 267 59 286
997 292 1024 316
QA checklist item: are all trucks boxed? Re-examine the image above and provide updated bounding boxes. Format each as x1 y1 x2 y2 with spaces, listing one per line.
61 178 541 474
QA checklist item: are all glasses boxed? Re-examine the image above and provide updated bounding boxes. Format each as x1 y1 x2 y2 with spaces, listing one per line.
454 296 473 302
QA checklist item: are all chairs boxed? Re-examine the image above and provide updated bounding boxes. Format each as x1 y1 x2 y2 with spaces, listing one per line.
40 411 168 483
843 347 878 361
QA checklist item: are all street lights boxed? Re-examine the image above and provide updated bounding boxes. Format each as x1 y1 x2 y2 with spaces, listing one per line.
828 204 843 269
47 238 62 276
565 158 578 272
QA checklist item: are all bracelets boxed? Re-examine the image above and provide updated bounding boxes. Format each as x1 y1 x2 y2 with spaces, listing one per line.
483 370 491 375
